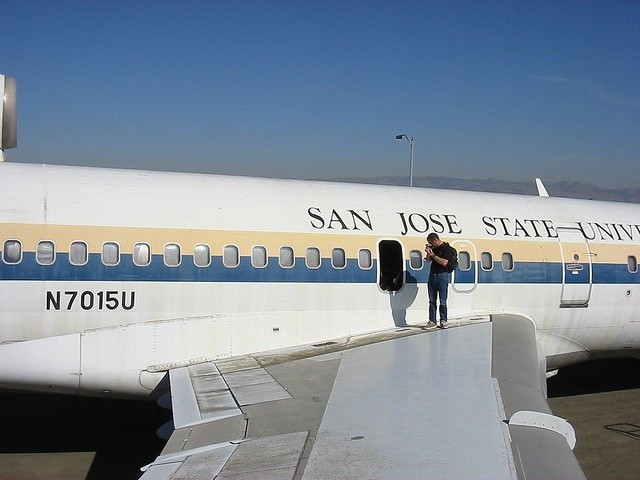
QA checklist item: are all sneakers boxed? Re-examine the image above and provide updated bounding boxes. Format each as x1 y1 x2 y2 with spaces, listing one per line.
440 320 448 329
421 320 438 330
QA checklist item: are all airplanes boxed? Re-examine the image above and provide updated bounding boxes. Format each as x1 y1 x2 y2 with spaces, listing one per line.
0 75 639 480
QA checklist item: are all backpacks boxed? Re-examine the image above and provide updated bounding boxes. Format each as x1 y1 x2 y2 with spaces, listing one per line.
447 242 458 273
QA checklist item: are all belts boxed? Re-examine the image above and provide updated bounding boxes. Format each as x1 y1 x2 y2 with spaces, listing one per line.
431 273 449 276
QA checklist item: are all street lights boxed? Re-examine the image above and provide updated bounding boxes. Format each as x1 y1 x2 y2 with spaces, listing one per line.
393 133 416 188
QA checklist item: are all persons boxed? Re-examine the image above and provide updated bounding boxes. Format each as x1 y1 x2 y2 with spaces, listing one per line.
423 233 449 329
